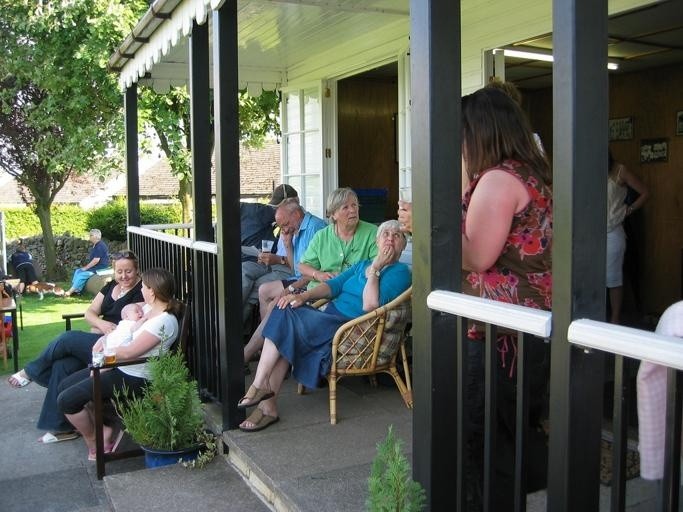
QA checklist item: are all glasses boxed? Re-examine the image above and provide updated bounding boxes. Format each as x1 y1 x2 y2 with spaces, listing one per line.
110 251 136 260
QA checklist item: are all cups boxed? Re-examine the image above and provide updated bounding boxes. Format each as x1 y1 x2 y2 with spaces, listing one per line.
92 352 103 367
103 352 115 364
260 240 274 253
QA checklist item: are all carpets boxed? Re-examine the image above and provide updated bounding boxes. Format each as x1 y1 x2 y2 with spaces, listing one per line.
540 430 641 487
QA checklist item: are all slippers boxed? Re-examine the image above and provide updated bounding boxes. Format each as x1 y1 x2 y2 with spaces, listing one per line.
5 371 34 389
87 429 125 461
37 432 80 444
238 408 279 433
237 383 275 408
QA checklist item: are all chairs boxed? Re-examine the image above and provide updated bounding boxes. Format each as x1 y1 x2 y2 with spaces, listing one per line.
295 282 413 426
0 314 8 371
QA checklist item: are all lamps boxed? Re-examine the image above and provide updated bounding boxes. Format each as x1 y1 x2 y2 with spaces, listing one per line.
490 43 620 73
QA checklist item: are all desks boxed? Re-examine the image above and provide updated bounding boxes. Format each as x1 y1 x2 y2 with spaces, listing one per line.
0 277 24 332
0 297 20 373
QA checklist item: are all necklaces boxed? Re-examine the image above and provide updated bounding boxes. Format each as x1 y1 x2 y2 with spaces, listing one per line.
118 279 137 298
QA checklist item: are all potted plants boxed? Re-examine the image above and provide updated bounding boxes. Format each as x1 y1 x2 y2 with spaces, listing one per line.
108 323 220 472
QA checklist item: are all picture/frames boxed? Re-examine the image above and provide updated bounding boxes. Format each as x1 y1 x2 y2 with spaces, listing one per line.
608 116 635 142
635 136 669 164
674 112 682 137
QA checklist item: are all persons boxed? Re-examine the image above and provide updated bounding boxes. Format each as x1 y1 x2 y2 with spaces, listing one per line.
62 229 110 299
57 268 180 463
100 303 150 355
8 249 147 445
11 246 40 298
0 282 12 299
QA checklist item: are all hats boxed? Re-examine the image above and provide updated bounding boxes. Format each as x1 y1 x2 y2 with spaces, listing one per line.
266 183 299 208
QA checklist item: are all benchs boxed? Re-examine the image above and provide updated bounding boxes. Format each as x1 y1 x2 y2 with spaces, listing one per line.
60 294 192 481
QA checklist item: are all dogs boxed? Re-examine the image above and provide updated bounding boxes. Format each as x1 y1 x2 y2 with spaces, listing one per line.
27 282 65 301
